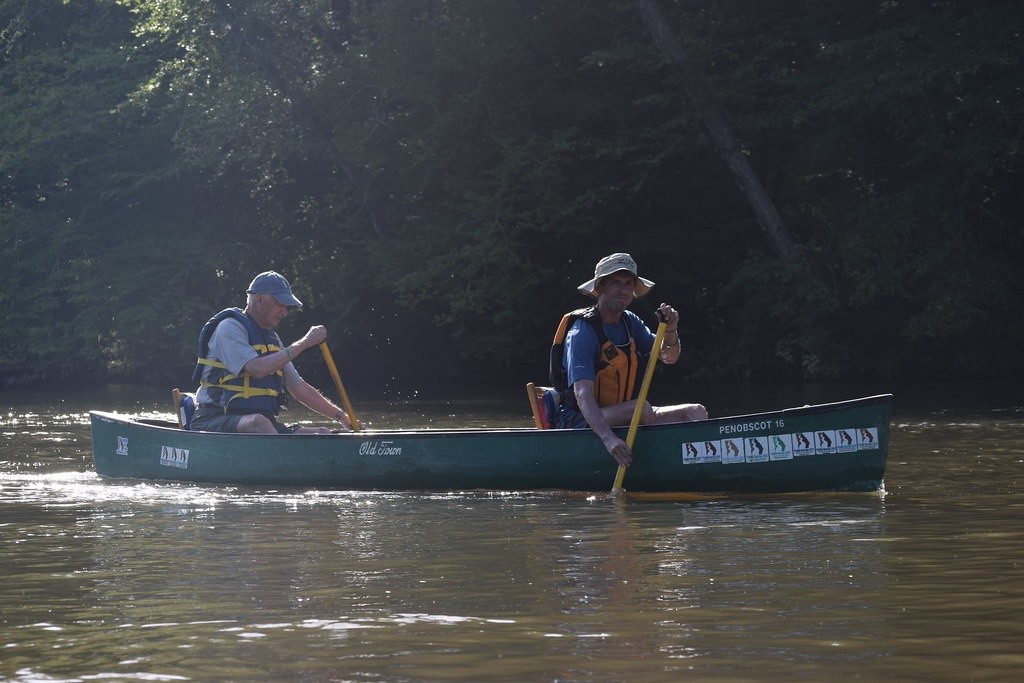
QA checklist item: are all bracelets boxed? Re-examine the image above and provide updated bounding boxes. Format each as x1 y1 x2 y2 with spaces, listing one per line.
665 327 677 333
334 410 344 422
662 338 678 351
284 348 294 361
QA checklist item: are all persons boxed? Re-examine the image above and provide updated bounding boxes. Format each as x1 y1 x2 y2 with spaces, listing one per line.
554 253 708 468
190 271 364 434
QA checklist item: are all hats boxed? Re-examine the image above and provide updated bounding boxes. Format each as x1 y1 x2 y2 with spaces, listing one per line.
577 253 655 299
247 270 304 309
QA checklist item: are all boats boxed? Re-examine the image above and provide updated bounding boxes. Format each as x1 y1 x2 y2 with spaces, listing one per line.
90 394 895 494
